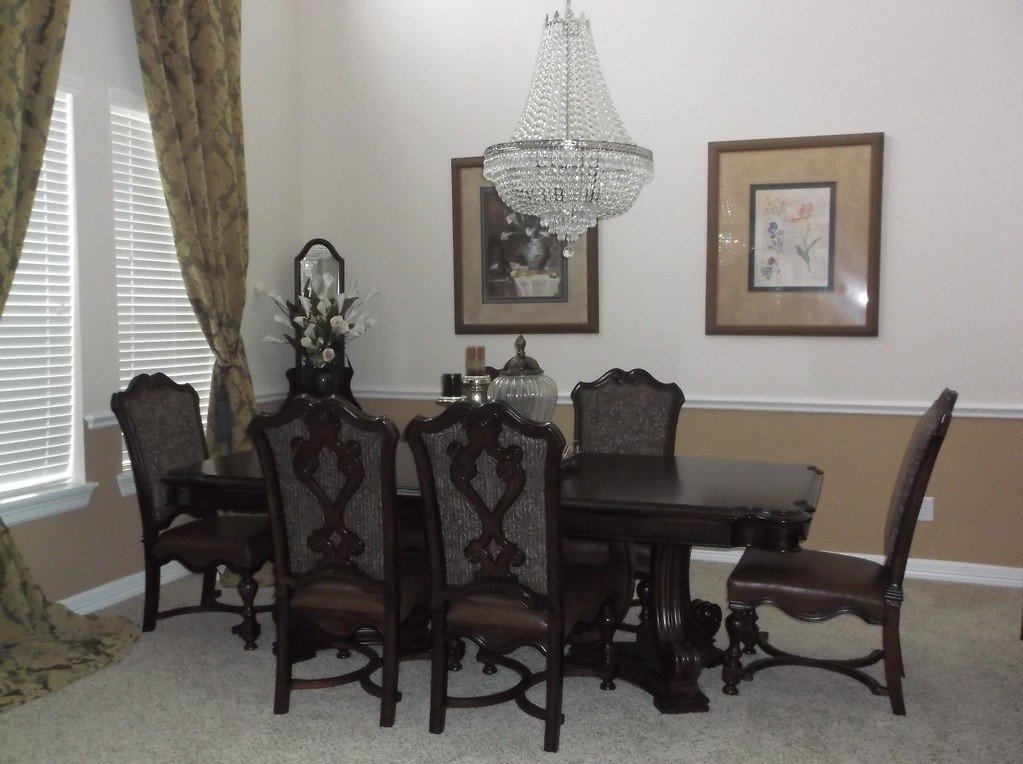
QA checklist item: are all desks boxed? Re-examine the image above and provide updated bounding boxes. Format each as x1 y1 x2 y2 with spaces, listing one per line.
157 440 823 716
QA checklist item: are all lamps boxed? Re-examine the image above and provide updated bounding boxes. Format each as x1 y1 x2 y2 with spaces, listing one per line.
443 374 461 397
483 0 654 257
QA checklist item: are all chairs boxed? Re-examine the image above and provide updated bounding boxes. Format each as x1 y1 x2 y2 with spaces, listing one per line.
571 368 685 579
110 372 271 650
721 387 957 716
404 399 622 753
247 394 433 727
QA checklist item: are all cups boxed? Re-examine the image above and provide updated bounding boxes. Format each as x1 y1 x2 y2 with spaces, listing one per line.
442 373 463 397
466 346 486 376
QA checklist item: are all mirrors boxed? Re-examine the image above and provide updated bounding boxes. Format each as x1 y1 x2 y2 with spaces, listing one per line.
295 238 344 364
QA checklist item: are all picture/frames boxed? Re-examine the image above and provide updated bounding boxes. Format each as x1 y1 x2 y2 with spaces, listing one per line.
451 156 599 334
705 132 884 337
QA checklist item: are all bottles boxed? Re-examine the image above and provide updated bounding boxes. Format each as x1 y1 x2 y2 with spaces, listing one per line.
487 336 557 423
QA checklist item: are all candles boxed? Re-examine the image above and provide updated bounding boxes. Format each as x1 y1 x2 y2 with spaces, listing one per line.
466 345 485 376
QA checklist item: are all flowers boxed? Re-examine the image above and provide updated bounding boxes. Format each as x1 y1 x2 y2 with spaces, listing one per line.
261 272 381 369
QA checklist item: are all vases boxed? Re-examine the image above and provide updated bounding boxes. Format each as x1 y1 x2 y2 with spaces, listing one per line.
284 366 362 411
